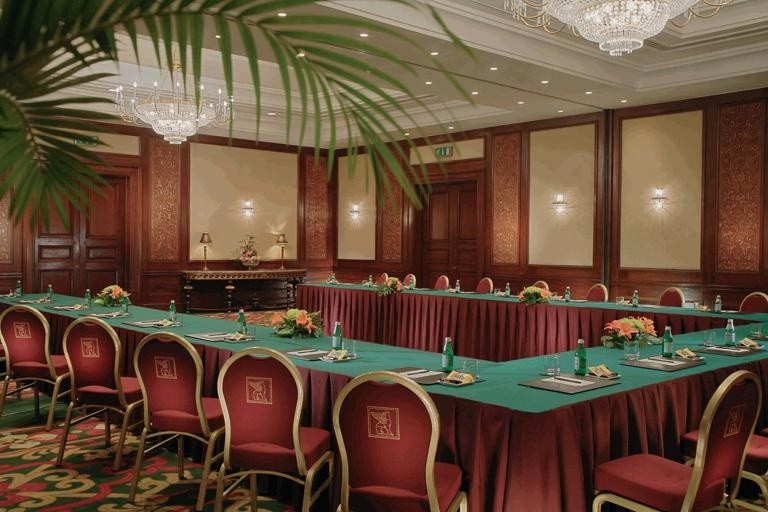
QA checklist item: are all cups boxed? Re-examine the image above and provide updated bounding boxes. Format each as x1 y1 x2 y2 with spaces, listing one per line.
342 340 357 359
170 314 183 327
614 296 625 305
543 353 560 376
702 330 714 347
623 341 639 362
748 321 762 338
461 357 481 381
243 325 256 340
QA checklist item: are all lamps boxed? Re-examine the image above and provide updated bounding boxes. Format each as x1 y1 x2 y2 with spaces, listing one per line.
276 233 288 270
200 232 212 270
107 46 236 144
508 0 734 56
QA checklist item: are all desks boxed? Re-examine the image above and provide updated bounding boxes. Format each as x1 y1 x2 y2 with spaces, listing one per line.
182 268 306 313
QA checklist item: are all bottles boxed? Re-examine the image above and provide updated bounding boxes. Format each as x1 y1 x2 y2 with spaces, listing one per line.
632 290 638 307
120 294 136 319
15 279 25 300
236 307 246 332
441 337 456 374
330 320 342 350
84 287 96 310
564 287 571 303
662 326 674 357
168 300 176 320
46 285 57 306
574 339 587 376
714 294 721 312
723 318 736 345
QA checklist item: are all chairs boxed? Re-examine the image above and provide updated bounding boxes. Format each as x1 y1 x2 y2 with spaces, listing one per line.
0 274 767 510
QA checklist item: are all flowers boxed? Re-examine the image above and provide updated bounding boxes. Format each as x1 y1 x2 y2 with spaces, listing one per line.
240 235 260 263
